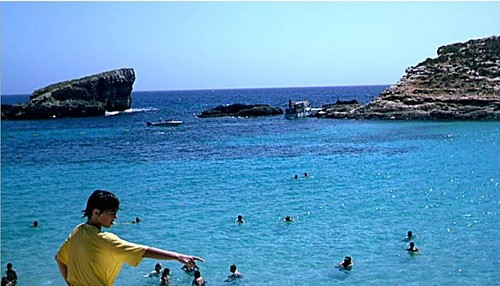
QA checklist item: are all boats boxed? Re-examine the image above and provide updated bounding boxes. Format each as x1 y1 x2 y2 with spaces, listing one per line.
146 119 184 128
284 100 313 120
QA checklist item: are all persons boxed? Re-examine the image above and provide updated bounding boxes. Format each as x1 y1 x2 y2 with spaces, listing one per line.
159 268 170 286
149 263 162 278
181 264 199 271
54 190 205 286
406 242 419 252
228 265 244 278
3 262 18 286
406 231 415 240
192 270 205 286
342 256 352 268
